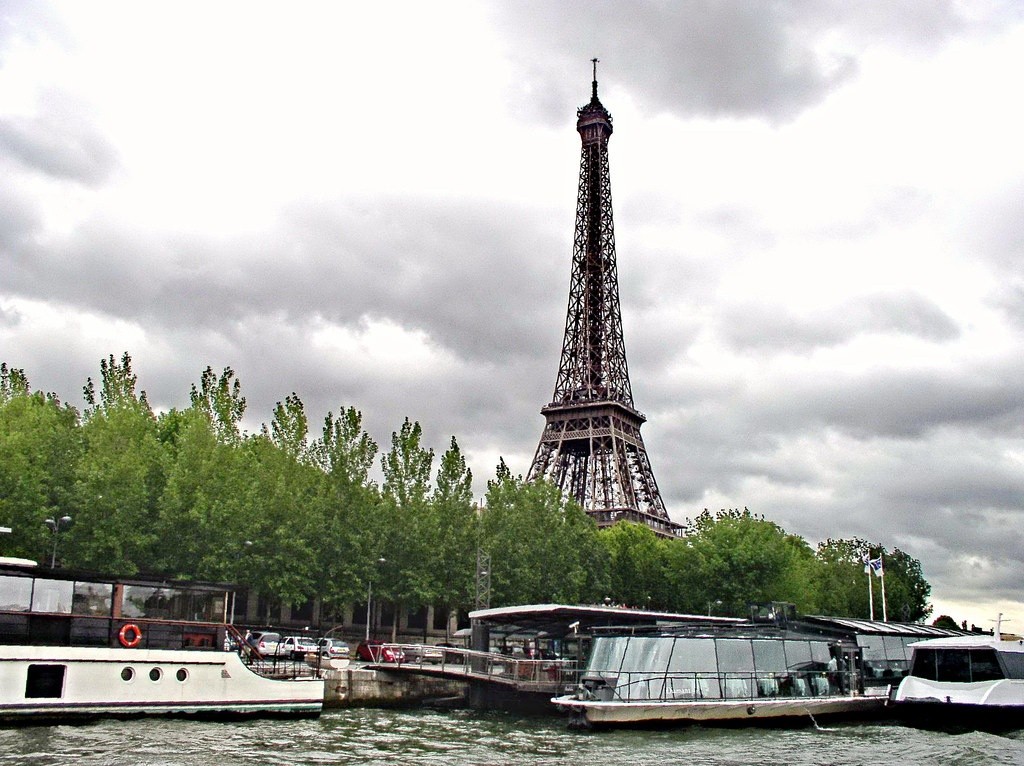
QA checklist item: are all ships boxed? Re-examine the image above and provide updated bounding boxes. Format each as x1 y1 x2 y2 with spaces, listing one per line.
0 554 1022 734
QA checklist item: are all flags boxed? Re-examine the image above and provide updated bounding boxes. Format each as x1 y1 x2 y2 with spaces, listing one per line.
862 553 870 574
869 557 882 577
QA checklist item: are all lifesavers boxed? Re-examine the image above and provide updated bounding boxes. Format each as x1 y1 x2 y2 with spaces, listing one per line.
120 623 142 648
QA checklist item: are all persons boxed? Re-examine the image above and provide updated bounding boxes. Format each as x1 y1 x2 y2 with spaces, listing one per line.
827 656 838 681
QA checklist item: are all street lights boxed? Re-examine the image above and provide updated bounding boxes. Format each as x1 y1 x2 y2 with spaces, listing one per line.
45 514 72 568
